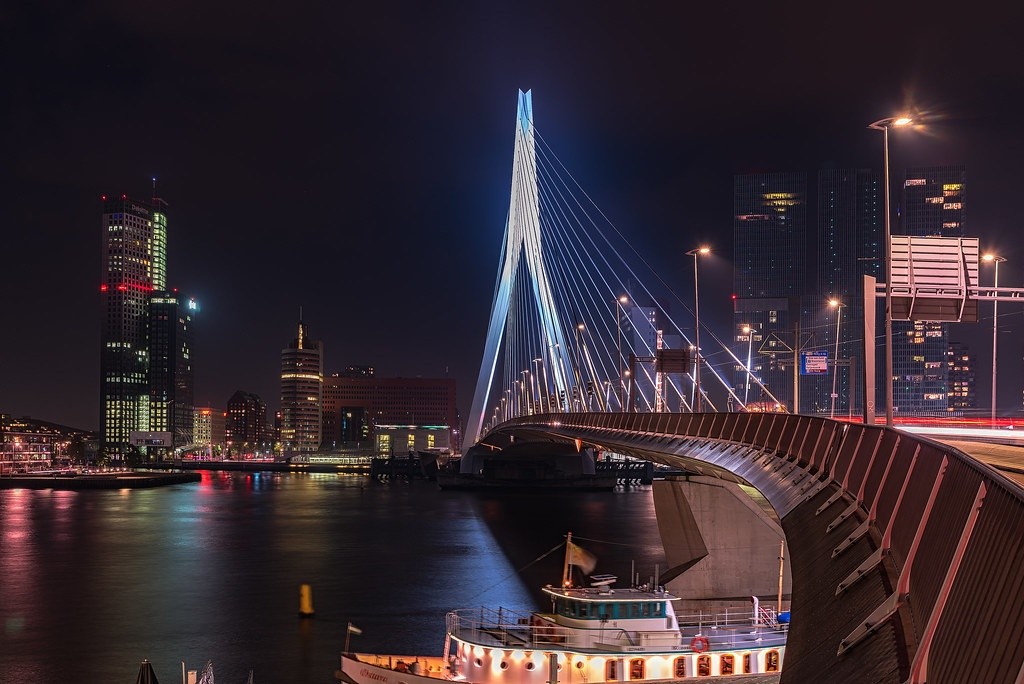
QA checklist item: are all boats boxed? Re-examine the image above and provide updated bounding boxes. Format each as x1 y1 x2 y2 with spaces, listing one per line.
335 531 790 684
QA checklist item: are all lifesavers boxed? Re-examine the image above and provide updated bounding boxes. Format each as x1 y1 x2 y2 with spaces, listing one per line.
690 637 708 653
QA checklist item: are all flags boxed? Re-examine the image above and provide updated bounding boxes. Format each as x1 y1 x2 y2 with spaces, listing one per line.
567 542 598 576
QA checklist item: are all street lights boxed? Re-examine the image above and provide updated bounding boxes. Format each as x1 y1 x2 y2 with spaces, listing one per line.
831 300 847 418
484 389 510 435
533 358 542 414
685 247 711 411
574 325 585 413
743 327 758 406
511 380 520 417
548 343 560 413
612 296 627 413
521 370 529 415
982 251 1008 429
869 116 912 427
